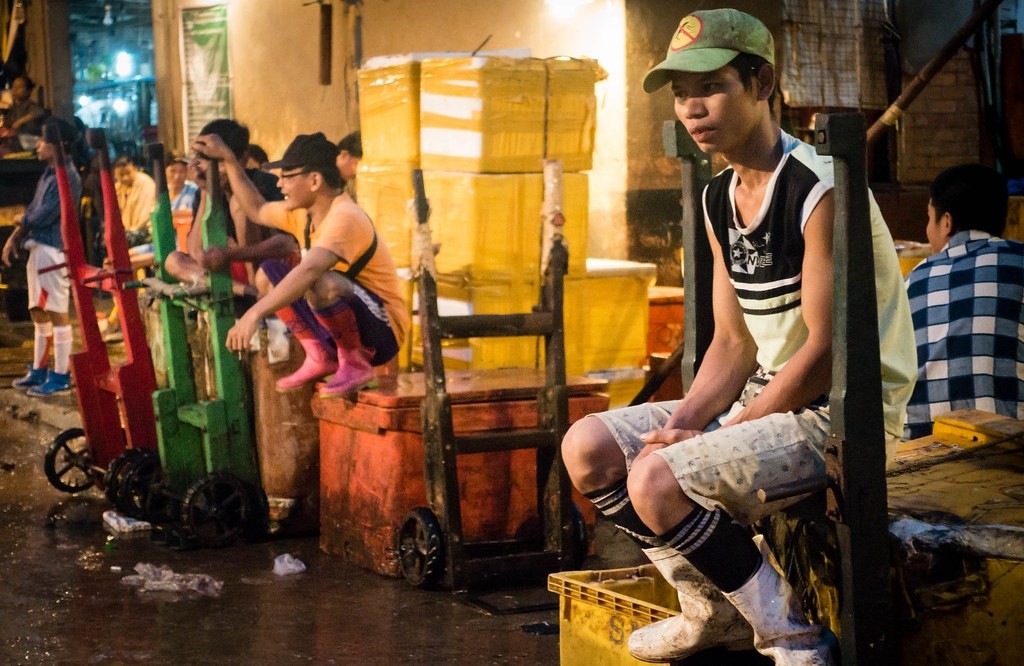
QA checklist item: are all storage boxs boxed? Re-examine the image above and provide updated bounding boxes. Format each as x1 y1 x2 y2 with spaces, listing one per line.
149 52 683 580
548 409 1024 666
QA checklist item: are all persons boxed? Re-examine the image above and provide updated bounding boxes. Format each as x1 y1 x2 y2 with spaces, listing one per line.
902 162 1023 440
0 75 410 398
561 8 919 666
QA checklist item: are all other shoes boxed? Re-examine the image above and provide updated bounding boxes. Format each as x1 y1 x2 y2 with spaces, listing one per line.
98 319 123 342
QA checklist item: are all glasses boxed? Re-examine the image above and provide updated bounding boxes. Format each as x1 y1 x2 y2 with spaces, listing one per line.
278 169 311 187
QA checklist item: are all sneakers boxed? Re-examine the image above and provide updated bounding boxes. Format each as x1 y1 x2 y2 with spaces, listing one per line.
27 370 72 397
12 365 48 389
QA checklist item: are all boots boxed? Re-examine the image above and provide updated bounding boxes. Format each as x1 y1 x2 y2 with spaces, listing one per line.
310 299 378 398
720 534 841 666
274 305 339 392
627 544 755 663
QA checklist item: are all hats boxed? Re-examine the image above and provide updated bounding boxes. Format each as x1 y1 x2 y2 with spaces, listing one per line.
643 9 774 93
264 133 339 168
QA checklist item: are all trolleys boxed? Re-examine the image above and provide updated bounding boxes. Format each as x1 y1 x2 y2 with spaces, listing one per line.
662 105 920 666
42 120 272 561
394 158 593 617
